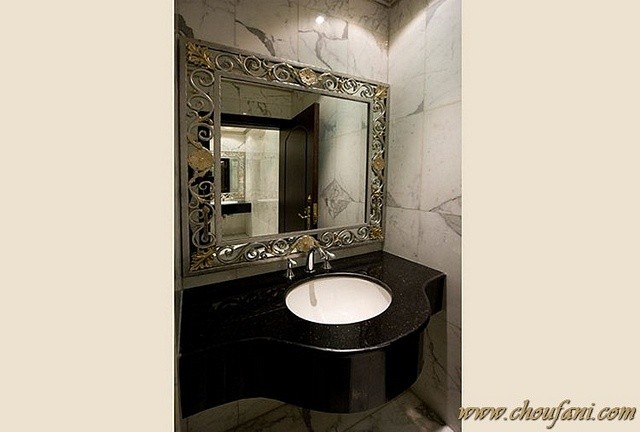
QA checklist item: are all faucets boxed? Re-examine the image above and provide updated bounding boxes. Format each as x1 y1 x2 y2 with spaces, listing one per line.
304 246 329 273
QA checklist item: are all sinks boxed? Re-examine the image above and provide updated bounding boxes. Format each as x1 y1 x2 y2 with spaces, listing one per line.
284 272 393 325
210 200 239 205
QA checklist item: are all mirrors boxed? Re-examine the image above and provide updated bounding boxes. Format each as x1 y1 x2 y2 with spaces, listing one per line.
177 34 390 279
211 150 246 202
